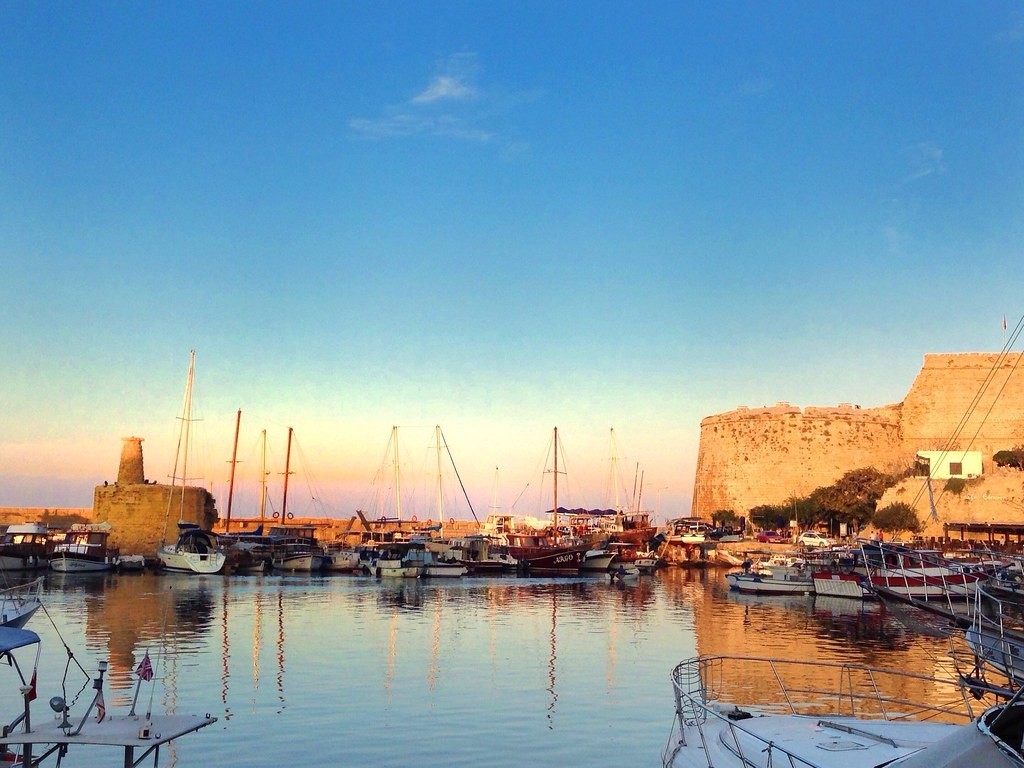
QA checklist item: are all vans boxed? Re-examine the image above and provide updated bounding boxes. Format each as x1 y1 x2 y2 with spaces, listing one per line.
712 526 733 534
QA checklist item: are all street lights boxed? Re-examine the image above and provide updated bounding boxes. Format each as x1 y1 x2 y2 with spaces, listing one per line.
752 516 764 535
656 486 669 526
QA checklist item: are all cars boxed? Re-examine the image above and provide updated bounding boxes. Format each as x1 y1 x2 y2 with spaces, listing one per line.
756 531 784 543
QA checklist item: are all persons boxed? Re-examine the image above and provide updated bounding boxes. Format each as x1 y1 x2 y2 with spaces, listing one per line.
610 565 625 580
869 530 883 541
680 521 687 537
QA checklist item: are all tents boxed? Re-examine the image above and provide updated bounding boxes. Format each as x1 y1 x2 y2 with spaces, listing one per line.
545 507 617 524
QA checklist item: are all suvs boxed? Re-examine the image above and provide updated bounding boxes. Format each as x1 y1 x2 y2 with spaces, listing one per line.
798 532 837 547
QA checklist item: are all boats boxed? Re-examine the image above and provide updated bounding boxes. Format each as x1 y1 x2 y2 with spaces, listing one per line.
48 525 121 571
0 523 51 570
145 350 1024 768
